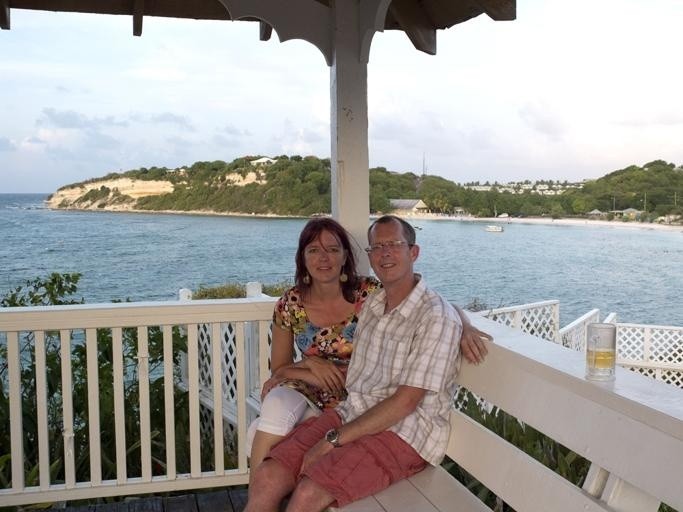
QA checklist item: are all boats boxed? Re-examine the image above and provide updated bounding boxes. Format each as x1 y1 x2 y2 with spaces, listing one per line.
484 225 504 232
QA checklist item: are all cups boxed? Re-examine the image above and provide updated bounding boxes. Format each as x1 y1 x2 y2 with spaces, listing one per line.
585 322 617 382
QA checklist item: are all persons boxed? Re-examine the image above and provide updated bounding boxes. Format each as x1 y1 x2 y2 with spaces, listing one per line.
241 214 461 511
248 214 494 492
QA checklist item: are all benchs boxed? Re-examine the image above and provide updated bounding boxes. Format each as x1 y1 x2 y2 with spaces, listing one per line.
325 332 683 511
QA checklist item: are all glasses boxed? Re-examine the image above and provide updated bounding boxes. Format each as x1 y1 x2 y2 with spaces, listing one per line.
366 241 406 252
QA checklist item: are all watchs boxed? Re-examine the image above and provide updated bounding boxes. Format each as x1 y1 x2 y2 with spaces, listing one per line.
324 427 341 451
